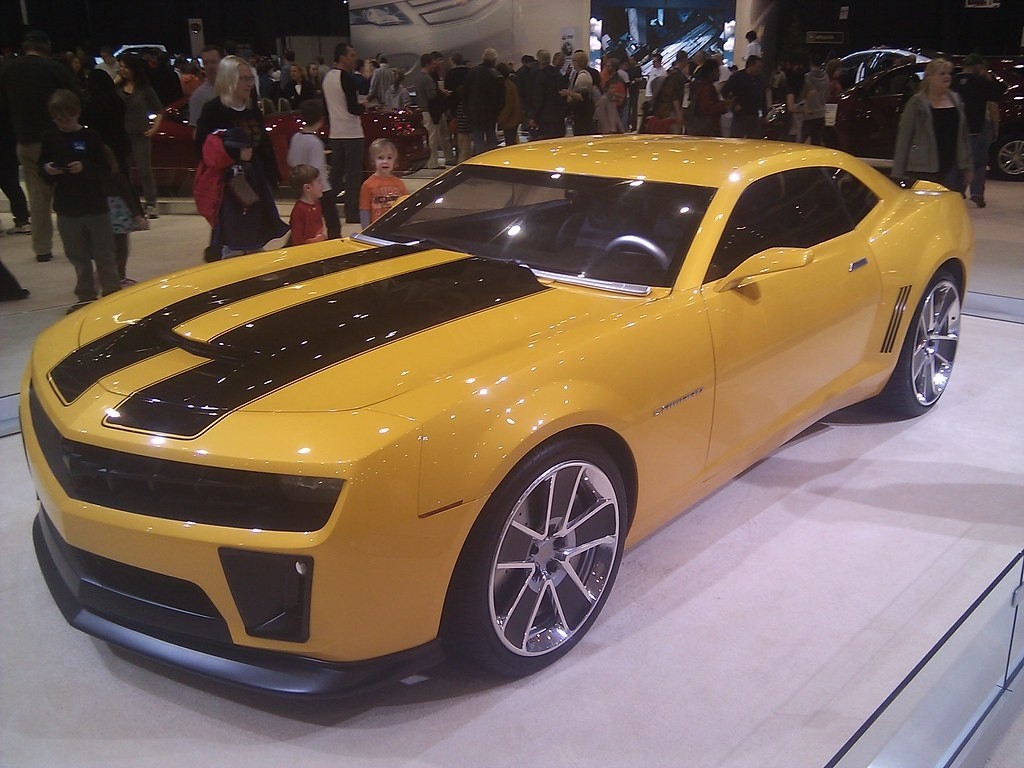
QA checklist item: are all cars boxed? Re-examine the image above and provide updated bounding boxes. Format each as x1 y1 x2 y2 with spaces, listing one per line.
756 56 1024 182
830 50 931 92
136 96 432 202
16 133 975 711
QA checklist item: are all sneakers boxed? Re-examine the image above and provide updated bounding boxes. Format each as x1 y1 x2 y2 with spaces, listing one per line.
7 223 31 235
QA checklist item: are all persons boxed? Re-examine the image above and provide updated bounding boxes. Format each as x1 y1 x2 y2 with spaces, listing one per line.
592 80 625 134
890 59 975 196
359 139 406 229
0 25 1001 305
289 164 328 245
384 69 411 109
37 90 122 315
287 99 342 240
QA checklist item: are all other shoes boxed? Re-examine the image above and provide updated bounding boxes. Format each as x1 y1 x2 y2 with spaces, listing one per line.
67 302 86 314
971 195 985 207
120 278 137 287
37 253 51 261
147 204 158 218
345 213 360 223
3 288 30 299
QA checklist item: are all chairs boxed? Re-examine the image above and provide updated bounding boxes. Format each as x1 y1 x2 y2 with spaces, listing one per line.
555 188 651 268
278 97 292 113
261 97 277 116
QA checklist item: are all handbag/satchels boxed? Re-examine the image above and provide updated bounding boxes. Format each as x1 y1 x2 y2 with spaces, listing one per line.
684 83 716 132
107 184 151 234
